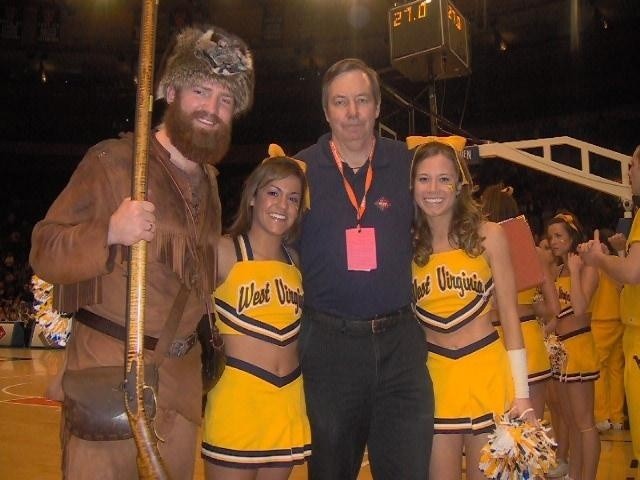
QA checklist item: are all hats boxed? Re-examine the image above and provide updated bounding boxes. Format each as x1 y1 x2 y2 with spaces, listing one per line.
155 25 253 114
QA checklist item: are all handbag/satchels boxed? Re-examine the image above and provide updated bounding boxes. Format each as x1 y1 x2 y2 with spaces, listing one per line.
61 365 158 441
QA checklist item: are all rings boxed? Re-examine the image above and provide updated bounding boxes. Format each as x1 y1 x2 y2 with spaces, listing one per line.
148 224 153 231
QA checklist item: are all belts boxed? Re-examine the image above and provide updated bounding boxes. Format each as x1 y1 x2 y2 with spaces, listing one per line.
301 304 413 335
76 306 201 358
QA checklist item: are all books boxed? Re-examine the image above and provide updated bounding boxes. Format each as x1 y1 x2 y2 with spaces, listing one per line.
497 215 545 291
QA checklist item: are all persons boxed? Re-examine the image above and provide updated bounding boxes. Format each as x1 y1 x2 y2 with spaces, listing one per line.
0 208 55 348
290 59 435 480
407 134 559 480
29 23 255 480
476 144 640 480
200 144 312 480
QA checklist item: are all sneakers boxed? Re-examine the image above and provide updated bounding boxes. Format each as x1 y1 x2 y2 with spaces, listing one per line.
543 462 568 477
